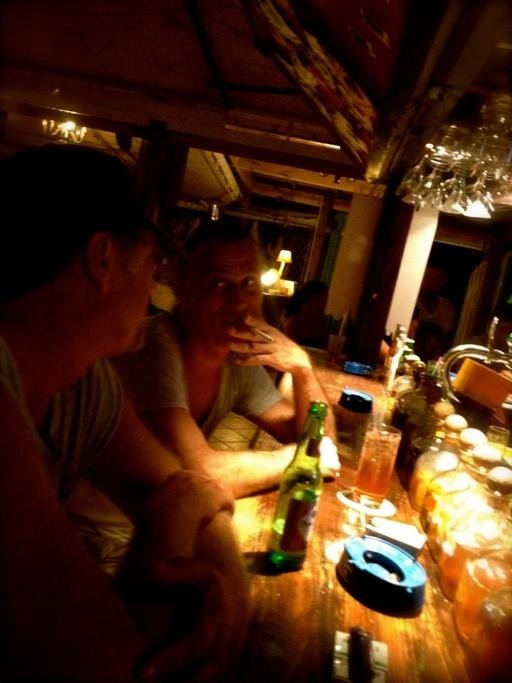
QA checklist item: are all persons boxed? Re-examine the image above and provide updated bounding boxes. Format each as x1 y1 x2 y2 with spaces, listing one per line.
279 278 340 350
414 320 448 363
0 144 257 683
257 219 284 333
116 218 347 500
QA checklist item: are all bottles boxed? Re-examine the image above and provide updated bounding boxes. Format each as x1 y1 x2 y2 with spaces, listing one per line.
262 398 328 572
378 323 512 683
333 388 372 446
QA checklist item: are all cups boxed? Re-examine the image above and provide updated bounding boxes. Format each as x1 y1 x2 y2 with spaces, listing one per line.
352 427 403 509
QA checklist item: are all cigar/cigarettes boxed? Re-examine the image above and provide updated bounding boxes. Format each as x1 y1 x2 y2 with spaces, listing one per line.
247 326 274 342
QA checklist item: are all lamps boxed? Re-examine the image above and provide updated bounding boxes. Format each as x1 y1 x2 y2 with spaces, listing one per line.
37 106 91 148
275 248 294 276
